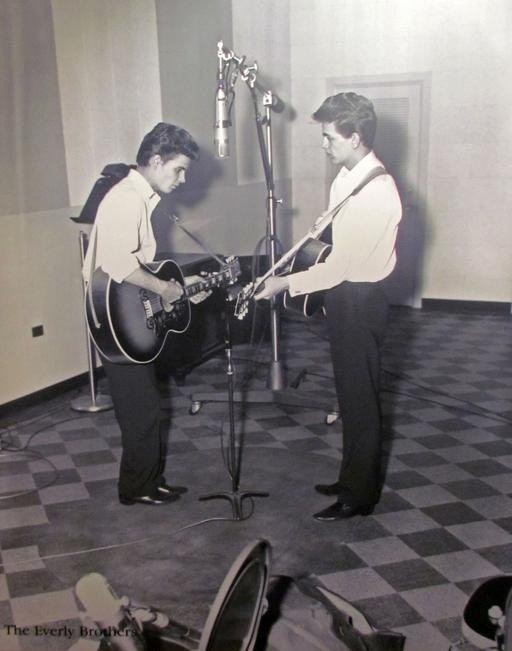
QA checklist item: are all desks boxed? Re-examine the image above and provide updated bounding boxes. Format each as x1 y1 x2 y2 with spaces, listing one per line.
154 252 234 386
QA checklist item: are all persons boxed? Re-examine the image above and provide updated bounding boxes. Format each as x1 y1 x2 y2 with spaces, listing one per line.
80 124 201 506
250 91 402 523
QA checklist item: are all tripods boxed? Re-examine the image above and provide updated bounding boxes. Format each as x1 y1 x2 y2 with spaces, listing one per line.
158 202 269 521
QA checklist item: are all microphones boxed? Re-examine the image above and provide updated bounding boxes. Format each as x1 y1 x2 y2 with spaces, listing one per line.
76 571 146 651
213 88 230 159
271 95 285 113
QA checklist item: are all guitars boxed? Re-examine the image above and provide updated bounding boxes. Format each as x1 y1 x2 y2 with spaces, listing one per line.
83 254 242 366
233 236 333 321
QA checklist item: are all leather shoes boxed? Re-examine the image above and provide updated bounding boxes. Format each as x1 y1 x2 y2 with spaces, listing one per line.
312 482 375 523
118 482 188 505
